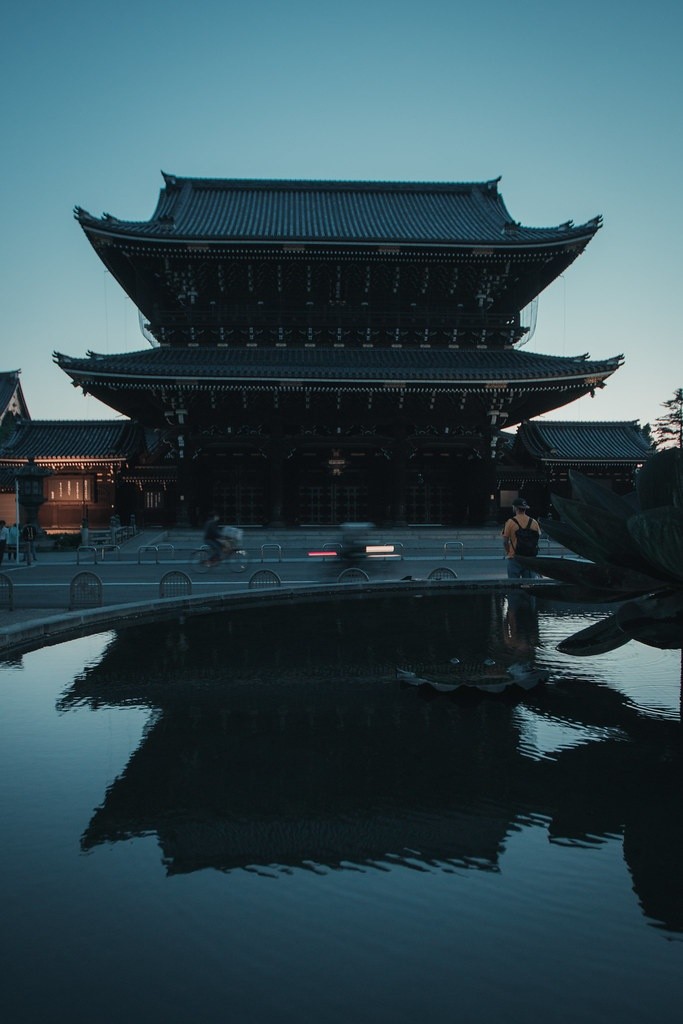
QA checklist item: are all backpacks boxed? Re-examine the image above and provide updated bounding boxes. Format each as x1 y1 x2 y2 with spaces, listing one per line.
24 526 34 541
508 516 540 558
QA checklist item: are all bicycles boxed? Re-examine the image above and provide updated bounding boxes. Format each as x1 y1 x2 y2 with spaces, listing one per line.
188 536 249 575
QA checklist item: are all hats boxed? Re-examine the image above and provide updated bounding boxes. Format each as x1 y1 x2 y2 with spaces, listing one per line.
511 497 530 508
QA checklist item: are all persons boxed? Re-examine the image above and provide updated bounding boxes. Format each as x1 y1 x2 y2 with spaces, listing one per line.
203 515 231 566
504 496 542 578
0 519 37 564
